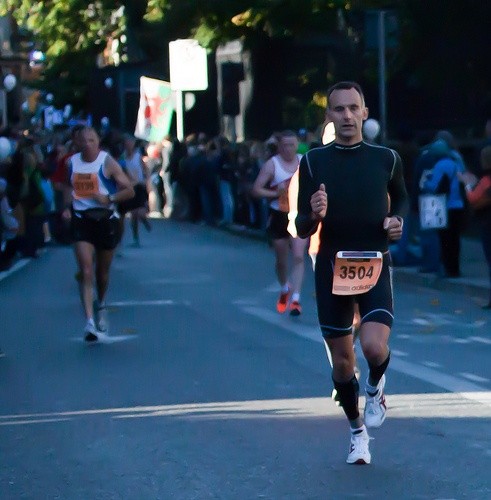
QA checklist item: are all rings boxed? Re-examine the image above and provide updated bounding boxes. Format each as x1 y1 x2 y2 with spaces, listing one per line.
317 202 319 206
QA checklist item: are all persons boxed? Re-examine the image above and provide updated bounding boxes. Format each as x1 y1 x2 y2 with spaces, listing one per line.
422 141 464 279
287 81 410 465
457 144 491 310
0 128 282 272
417 130 463 280
251 129 307 315
61 127 136 342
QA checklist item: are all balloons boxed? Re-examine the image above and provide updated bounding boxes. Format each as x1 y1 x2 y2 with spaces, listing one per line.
105 77 113 90
101 116 110 127
363 118 380 141
0 137 11 162
45 93 54 103
4 73 17 92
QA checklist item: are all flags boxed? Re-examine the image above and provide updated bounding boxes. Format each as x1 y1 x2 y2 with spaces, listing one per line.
133 75 173 143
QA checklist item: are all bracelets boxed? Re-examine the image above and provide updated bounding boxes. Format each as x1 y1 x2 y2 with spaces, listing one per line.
107 193 115 204
